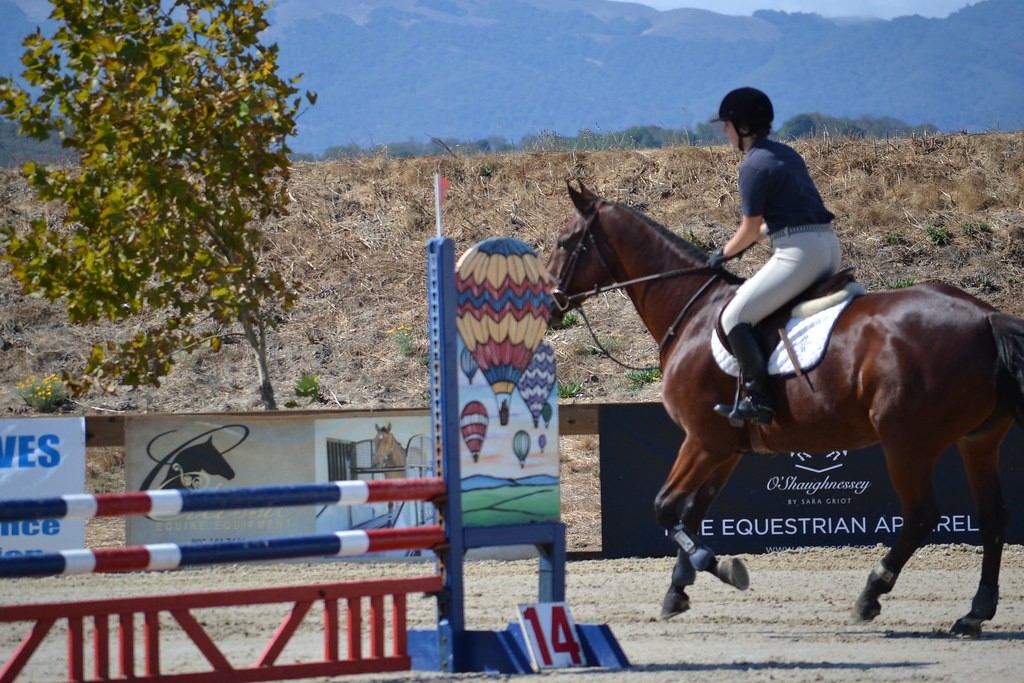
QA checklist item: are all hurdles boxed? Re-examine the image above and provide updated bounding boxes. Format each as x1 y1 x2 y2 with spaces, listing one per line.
0 236 636 683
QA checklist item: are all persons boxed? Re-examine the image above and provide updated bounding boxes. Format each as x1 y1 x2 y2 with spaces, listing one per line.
706 86 842 426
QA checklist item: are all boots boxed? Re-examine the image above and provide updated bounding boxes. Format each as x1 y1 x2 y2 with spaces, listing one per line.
714 321 772 428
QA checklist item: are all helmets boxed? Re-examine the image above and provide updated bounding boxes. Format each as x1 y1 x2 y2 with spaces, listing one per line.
709 87 774 138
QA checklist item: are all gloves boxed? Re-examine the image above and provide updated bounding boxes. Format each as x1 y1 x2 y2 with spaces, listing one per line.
707 248 728 267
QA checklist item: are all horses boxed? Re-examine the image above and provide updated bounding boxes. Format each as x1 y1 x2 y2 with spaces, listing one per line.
541 175 1024 638
